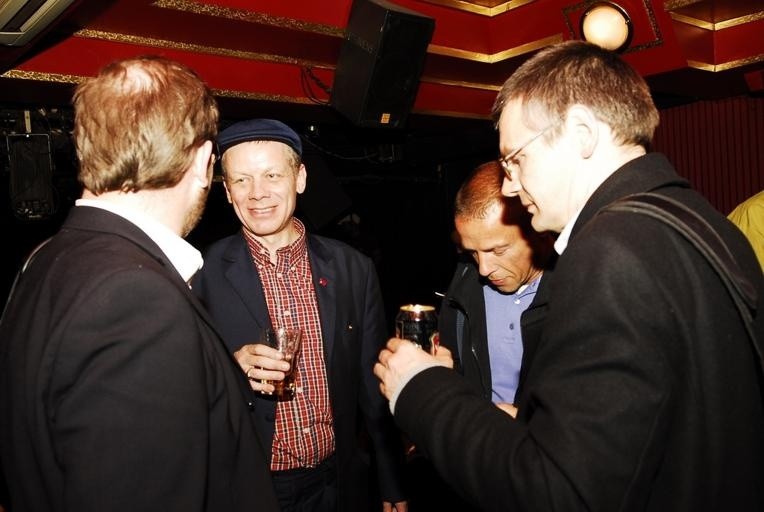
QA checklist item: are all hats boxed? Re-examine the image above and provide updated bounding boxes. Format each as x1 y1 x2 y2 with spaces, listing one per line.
218 119 303 156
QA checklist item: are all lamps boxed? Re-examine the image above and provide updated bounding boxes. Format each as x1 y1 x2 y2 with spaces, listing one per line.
562 0 664 55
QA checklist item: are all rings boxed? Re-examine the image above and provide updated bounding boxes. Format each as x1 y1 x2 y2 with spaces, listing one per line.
246 367 253 377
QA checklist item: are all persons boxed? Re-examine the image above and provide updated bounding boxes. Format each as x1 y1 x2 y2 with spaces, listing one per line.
372 39 761 511
0 55 280 511
187 118 411 512
436 159 559 422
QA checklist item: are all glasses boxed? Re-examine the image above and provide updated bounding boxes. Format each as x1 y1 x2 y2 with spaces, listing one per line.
498 116 562 181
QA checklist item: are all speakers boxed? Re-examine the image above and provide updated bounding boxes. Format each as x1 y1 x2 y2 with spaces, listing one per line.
5 133 55 220
329 0 435 134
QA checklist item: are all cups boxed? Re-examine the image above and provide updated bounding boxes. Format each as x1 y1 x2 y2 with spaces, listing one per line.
258 326 301 402
